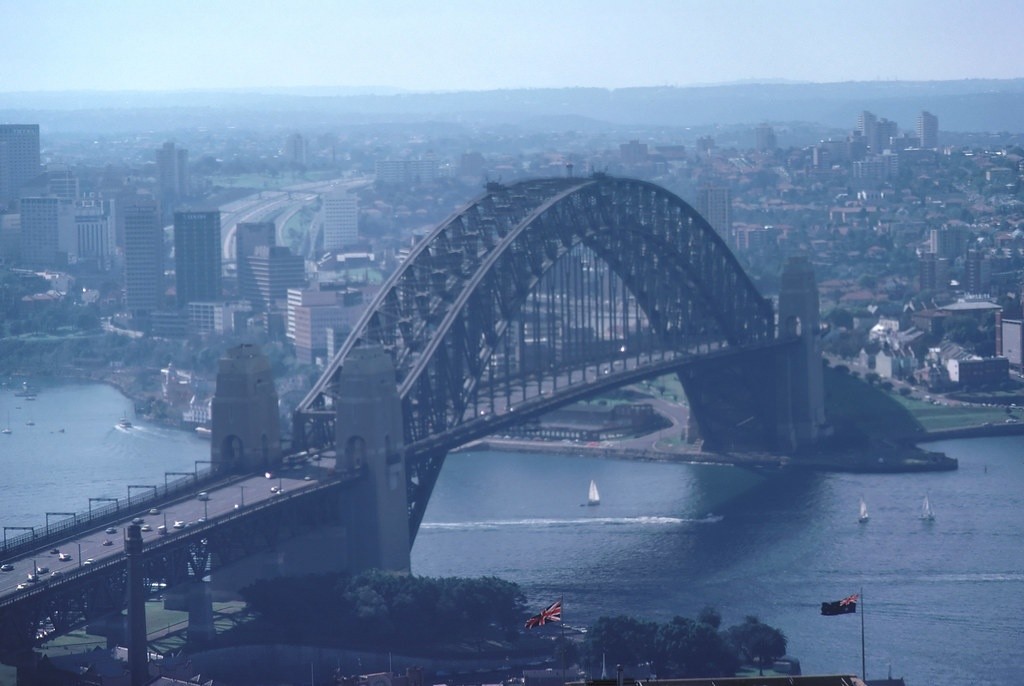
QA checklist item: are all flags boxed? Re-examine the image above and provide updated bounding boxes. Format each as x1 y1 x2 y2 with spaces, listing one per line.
820 593 858 616
524 600 561 630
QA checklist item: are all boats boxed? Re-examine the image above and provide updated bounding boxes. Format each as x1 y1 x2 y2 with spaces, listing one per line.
26 420 37 427
118 417 135 431
49 429 66 434
14 392 38 409
698 512 726 525
2 428 13 435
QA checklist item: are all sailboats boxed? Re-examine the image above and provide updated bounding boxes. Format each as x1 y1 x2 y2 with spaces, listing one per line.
588 479 601 506
858 496 871 524
916 490 938 522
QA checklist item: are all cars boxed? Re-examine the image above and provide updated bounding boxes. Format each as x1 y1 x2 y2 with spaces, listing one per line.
36 567 50 574
84 558 96 565
26 574 40 582
1 563 15 572
479 408 488 416
50 548 59 554
103 449 322 548
51 570 63 579
16 582 32 591
59 553 72 561
